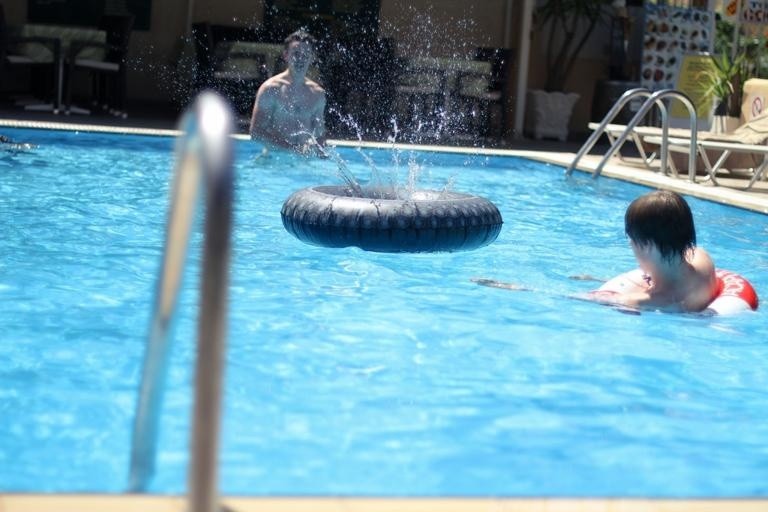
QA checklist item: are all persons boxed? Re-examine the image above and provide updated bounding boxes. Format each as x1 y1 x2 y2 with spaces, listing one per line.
250 30 327 155
468 189 718 312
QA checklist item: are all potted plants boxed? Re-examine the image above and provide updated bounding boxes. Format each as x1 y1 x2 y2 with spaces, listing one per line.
527 0 618 142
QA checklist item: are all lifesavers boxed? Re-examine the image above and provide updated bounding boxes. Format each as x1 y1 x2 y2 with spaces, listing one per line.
280 182 505 253
595 263 756 320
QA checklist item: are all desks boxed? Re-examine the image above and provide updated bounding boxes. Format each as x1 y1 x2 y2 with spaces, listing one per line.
230 39 288 80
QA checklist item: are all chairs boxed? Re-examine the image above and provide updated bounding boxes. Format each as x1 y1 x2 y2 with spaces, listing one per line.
191 20 270 115
0 14 137 119
587 109 768 190
375 38 512 141
